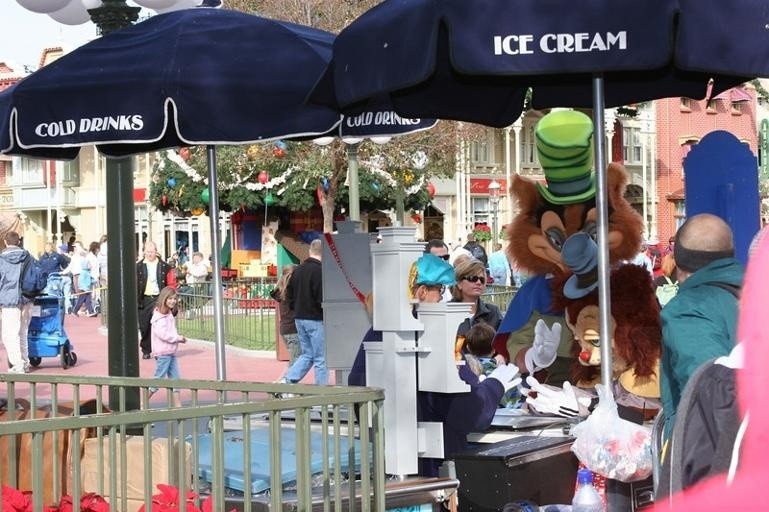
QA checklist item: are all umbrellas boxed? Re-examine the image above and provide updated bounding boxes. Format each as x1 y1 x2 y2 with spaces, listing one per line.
334 1 767 414
2 5 442 411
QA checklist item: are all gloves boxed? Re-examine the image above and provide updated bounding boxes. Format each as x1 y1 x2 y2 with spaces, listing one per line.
478 363 522 391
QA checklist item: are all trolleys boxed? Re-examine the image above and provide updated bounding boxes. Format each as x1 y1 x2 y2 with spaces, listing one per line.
23 294 78 371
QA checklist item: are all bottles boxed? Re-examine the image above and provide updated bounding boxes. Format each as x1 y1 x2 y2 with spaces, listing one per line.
572 469 603 511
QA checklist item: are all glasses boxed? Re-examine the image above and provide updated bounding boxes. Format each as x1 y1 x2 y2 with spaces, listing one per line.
464 275 486 284
436 254 449 261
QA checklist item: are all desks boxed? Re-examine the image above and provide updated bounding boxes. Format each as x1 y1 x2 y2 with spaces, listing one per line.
448 432 578 511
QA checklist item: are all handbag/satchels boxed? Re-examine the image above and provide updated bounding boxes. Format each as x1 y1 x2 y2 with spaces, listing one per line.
19 255 47 295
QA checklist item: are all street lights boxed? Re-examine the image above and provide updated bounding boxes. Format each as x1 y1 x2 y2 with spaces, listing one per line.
370 151 427 225
15 0 218 448
487 180 501 247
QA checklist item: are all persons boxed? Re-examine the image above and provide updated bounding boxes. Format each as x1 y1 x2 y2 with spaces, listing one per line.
0 231 109 374
345 213 742 493
271 239 329 401
136 242 208 410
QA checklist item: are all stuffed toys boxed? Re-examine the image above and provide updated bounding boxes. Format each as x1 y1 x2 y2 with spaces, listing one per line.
495 108 644 363
